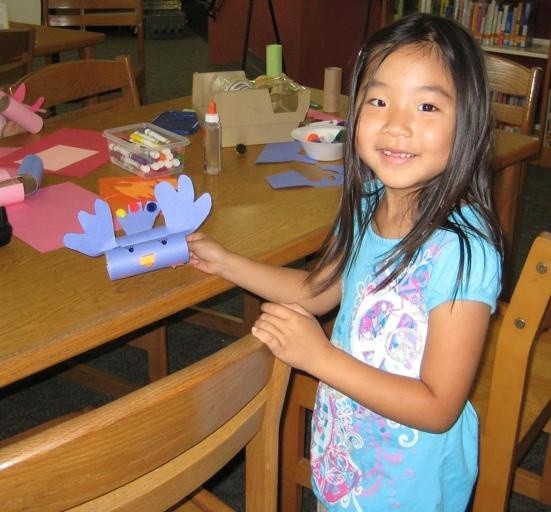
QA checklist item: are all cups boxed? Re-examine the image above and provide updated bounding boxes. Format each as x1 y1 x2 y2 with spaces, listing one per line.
212 71 252 93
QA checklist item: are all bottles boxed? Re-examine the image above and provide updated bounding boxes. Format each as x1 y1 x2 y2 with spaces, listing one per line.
202 99 224 176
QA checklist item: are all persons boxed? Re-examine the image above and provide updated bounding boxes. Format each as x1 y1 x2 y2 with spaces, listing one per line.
188 13 505 511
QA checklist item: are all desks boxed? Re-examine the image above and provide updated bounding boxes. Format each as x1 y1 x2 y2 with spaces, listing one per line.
1 68 539 400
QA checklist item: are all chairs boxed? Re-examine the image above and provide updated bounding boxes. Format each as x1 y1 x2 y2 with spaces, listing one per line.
0 329 292 512
1 1 150 120
280 233 551 512
484 51 542 247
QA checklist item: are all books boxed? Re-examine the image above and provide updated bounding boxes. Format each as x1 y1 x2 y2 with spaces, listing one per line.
389 0 534 48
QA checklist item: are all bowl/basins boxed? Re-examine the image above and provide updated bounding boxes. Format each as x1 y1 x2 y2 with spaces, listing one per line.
291 124 345 161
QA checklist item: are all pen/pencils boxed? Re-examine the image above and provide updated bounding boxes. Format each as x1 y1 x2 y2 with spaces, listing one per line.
181 108 196 112
310 100 321 110
108 128 184 179
